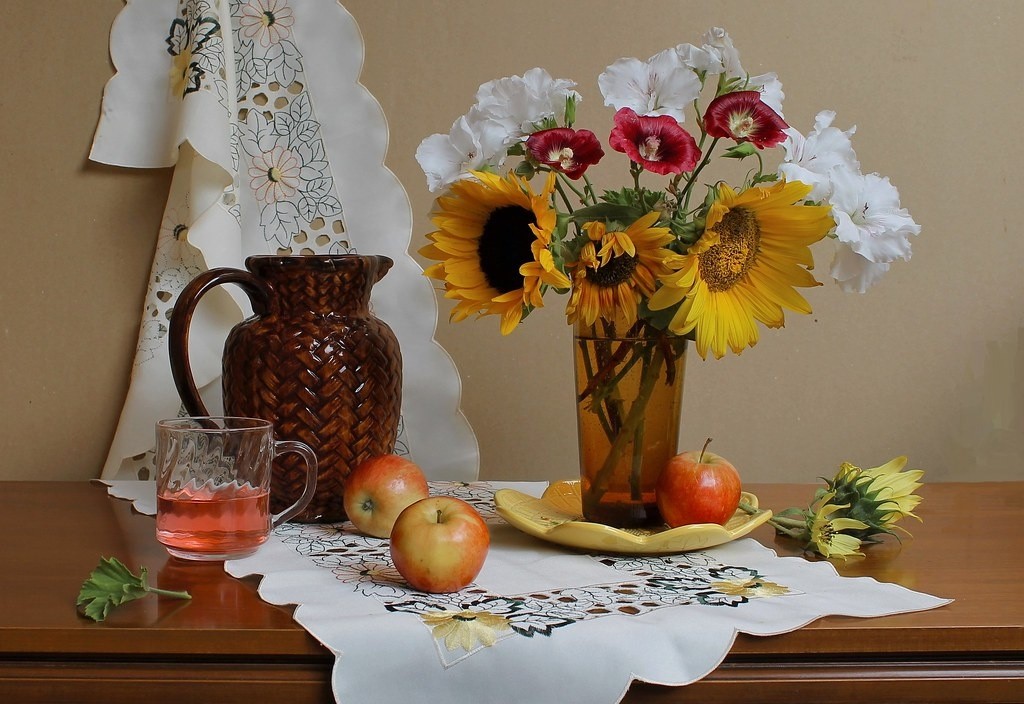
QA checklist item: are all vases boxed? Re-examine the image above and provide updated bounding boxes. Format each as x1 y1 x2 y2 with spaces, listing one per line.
573 302 687 526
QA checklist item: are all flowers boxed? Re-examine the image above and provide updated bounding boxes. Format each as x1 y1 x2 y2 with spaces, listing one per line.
735 456 928 563
414 25 924 501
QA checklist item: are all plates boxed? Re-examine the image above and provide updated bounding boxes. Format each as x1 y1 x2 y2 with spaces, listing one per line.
491 478 772 552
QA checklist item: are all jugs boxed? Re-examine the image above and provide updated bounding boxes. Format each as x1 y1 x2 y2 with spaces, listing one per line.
167 254 404 526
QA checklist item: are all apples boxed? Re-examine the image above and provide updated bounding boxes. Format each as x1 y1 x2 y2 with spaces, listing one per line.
655 438 742 529
389 496 489 593
344 454 429 538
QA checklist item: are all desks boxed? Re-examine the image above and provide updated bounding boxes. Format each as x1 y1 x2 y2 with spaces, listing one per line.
0 479 1024 704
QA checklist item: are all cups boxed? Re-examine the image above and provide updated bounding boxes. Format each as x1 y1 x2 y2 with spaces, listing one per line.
153 415 318 563
